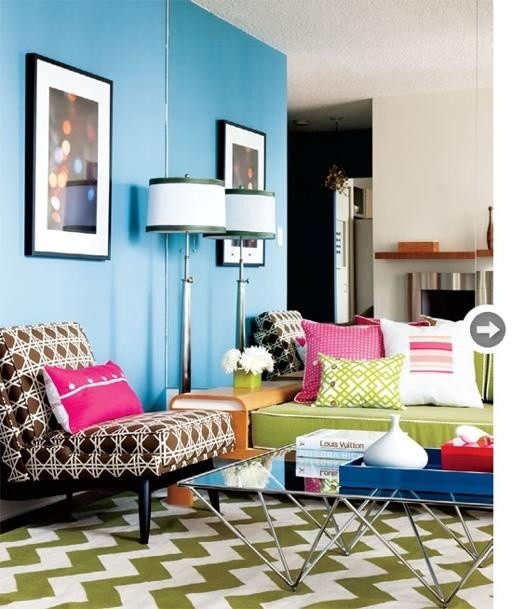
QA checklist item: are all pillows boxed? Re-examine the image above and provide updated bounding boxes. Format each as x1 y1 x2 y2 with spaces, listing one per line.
295 335 306 366
380 318 486 410
312 354 406 410
354 314 430 327
415 312 463 326
41 358 141 436
294 317 384 404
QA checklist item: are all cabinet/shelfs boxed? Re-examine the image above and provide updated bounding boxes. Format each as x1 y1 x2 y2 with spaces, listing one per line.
375 250 492 319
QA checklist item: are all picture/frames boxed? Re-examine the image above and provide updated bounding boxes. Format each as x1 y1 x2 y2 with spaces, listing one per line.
214 119 266 265
24 53 114 261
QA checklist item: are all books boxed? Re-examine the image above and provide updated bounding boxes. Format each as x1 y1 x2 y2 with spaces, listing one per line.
296 428 408 452
296 450 363 480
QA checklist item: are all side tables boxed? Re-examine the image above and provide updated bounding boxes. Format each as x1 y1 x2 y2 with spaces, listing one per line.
167 377 304 457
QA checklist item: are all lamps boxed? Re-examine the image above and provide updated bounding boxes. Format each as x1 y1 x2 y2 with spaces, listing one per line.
223 189 275 347
142 174 227 391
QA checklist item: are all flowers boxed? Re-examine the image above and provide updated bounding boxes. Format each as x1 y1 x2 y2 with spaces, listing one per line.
221 348 274 374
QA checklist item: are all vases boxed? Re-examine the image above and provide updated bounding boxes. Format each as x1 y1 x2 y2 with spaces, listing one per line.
361 415 429 470
233 373 262 392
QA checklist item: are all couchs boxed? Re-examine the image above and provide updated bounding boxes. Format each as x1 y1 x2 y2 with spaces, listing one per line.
0 321 237 544
249 310 495 450
252 310 306 382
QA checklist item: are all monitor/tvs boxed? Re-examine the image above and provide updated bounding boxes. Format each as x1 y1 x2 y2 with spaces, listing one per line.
420 288 475 322
